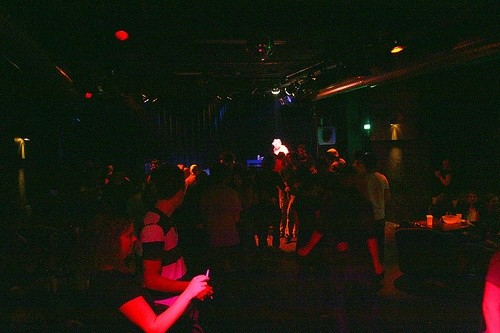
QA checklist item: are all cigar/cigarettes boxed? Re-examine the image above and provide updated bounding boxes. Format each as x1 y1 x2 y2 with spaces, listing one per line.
206 269 209 276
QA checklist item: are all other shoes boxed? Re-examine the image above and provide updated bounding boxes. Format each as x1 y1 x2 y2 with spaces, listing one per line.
283 233 293 243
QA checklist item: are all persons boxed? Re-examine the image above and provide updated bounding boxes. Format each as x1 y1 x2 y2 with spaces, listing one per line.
87 139 500 333
